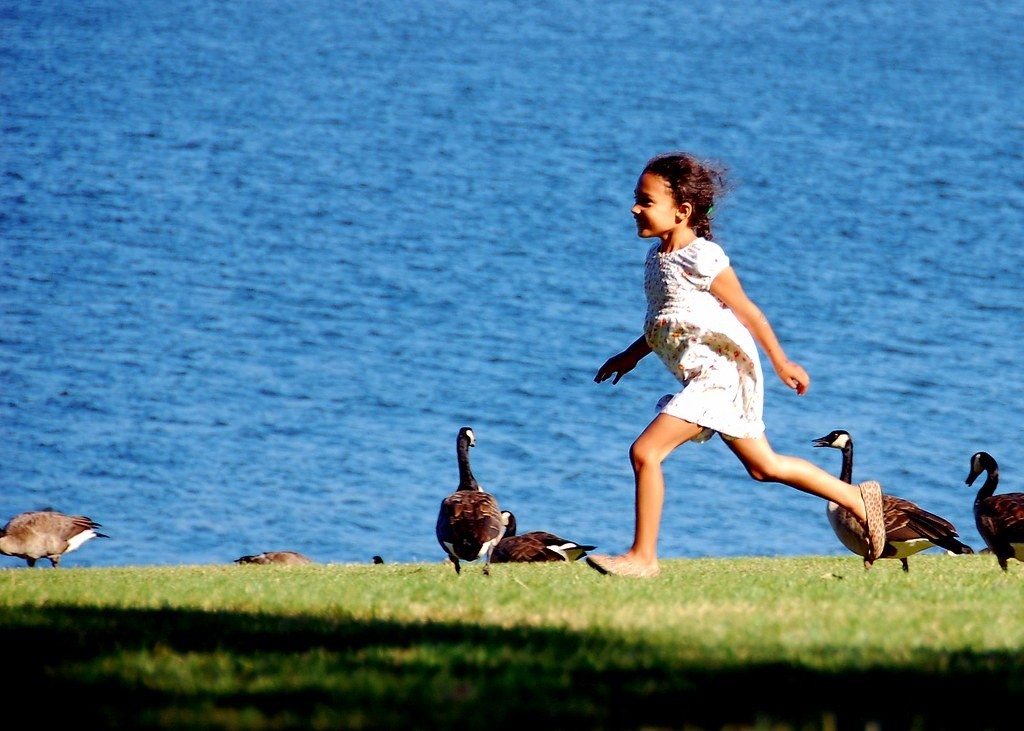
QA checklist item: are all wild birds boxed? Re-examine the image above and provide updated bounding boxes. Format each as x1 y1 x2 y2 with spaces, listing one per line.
0 507 111 570
812 430 976 576
233 551 312 567
436 426 599 577
965 452 1024 575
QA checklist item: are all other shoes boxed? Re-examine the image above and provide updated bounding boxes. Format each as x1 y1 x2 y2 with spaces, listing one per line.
859 479 886 563
585 554 661 578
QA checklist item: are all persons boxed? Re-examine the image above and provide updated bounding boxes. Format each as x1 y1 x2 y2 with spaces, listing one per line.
586 152 886 579
